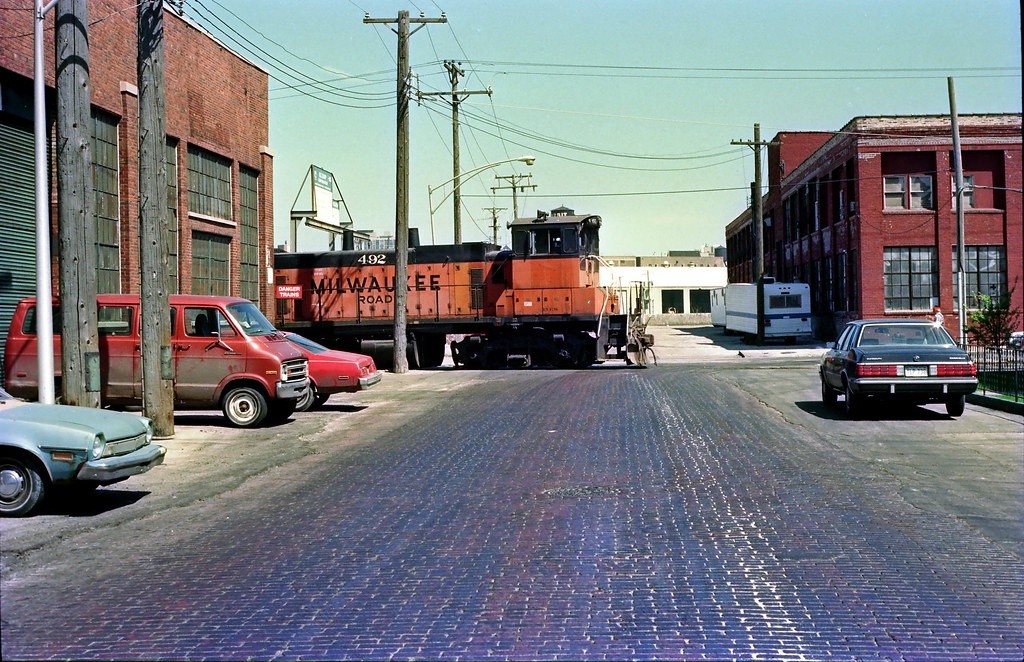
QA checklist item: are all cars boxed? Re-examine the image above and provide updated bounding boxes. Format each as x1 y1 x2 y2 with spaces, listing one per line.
216 324 382 412
1009 331 1024 353
819 317 979 420
0 387 168 519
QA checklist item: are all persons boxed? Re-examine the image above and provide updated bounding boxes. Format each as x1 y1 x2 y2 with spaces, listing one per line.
925 305 944 325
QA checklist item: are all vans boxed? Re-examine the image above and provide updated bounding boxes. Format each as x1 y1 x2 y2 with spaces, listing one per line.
5 293 311 429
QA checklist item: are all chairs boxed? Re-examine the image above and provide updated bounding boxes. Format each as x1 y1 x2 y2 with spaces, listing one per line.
860 339 879 345
906 338 926 343
195 314 212 336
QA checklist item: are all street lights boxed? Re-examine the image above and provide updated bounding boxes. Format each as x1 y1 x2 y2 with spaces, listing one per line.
427 156 535 246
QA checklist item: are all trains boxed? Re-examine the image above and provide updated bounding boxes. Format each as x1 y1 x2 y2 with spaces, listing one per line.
274 204 659 371
